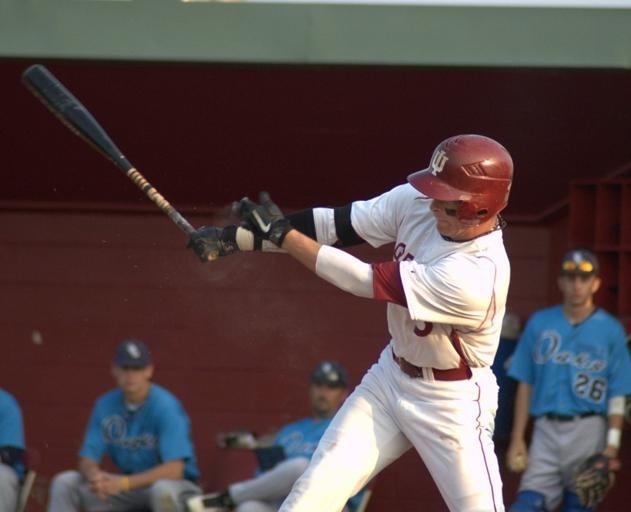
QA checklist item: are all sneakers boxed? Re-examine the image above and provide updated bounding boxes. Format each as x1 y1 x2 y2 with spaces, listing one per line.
183 488 233 512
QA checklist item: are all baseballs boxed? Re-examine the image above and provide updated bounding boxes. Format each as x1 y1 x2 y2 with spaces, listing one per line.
509 456 526 472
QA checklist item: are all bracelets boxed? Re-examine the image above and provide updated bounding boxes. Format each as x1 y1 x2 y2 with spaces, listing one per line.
606 428 622 450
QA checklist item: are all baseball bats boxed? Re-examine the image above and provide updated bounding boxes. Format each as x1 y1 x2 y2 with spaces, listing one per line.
21 62 221 262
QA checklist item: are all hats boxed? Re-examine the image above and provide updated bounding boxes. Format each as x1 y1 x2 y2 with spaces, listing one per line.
109 336 151 366
310 358 349 386
558 248 599 275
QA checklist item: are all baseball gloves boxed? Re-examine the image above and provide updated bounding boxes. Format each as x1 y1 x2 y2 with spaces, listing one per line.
571 451 619 508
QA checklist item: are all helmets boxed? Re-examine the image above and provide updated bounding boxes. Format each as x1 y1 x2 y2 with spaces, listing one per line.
406 133 517 225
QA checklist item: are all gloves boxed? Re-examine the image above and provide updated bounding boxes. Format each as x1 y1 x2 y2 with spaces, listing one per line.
184 225 234 263
232 191 293 250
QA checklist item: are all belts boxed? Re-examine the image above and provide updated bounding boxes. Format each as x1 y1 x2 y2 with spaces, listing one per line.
391 346 471 380
542 411 598 422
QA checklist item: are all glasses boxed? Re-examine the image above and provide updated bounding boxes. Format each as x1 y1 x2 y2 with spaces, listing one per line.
561 261 594 274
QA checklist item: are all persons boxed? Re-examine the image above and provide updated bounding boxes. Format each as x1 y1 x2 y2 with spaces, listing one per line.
186 135 516 512
186 359 374 512
505 248 630 512
0 390 27 512
46 338 202 512
